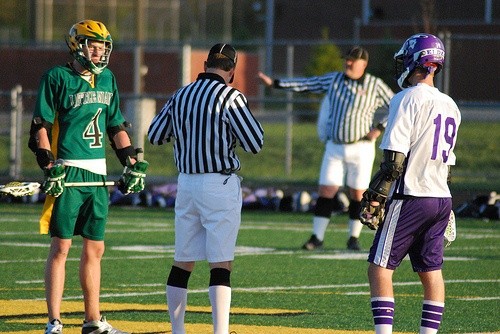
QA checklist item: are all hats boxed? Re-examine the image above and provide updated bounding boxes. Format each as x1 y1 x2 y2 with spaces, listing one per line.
340 45 368 60
208 43 238 84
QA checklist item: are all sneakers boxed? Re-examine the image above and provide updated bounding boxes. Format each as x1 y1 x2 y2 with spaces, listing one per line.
45 319 63 334
82 314 128 334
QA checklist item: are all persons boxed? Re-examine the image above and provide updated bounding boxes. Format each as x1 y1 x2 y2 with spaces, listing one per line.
27 19 149 334
147 43 264 334
355 33 461 334
257 45 395 251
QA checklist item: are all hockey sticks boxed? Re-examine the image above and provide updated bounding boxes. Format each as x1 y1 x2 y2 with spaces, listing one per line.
0 181 121 197
364 210 456 242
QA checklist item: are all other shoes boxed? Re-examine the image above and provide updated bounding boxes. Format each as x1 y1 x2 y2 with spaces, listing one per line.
302 234 323 251
347 236 359 250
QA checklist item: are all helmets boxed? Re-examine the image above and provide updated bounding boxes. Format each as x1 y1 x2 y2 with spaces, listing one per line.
393 32 445 90
65 19 112 75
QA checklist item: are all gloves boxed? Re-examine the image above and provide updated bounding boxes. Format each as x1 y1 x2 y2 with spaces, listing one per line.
118 156 150 195
39 164 65 197
